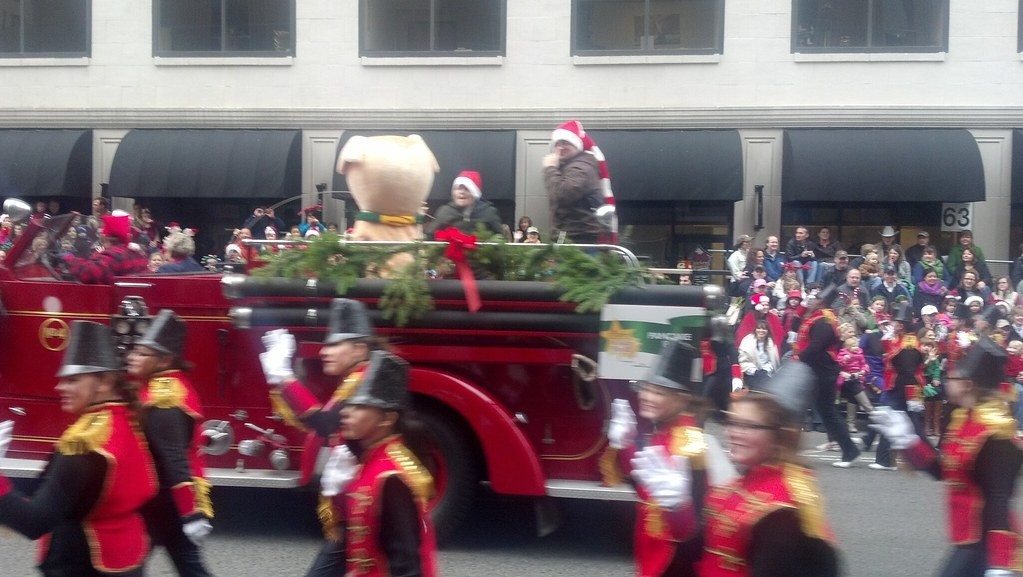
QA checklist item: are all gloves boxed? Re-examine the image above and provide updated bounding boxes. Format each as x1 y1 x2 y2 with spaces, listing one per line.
259 329 297 384
0 420 15 458
320 447 361 496
630 445 692 510
867 405 920 450
182 518 213 547
608 398 639 449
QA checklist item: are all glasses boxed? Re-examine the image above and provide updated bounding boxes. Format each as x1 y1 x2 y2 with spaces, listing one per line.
128 349 159 358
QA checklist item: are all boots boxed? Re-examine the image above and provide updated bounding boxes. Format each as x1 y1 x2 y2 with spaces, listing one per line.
854 391 874 412
846 402 858 433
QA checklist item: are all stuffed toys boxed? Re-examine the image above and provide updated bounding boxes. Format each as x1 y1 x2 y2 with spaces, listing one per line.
336 134 440 279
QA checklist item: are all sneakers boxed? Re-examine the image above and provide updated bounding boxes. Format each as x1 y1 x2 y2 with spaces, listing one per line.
833 454 861 468
816 437 864 452
868 463 898 471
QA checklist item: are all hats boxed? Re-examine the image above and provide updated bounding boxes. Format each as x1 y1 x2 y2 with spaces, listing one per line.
349 351 411 410
225 244 242 258
736 235 755 246
916 231 931 238
101 215 130 245
57 309 185 378
646 340 818 417
964 296 1011 314
306 228 320 238
548 118 584 151
526 225 539 234
785 290 804 302
835 249 848 258
921 305 938 316
891 303 914 322
751 264 770 305
877 226 900 238
324 298 374 346
451 171 483 201
956 334 1006 387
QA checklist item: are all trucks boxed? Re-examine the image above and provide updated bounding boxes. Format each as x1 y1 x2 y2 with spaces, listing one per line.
0 204 748 547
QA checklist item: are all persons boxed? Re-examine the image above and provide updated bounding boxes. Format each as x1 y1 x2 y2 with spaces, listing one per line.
259 298 439 577
0 308 214 577
426 171 506 280
679 226 1023 470
542 121 615 276
868 336 1023 577
0 197 354 285
501 216 541 278
607 339 840 577
